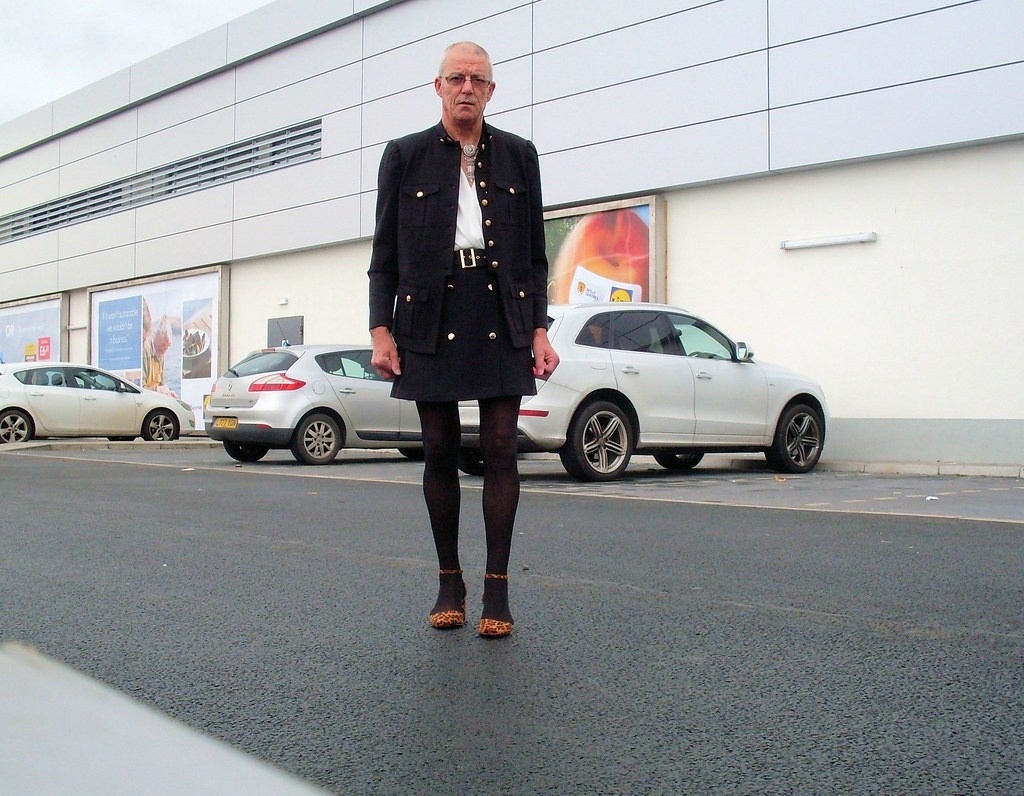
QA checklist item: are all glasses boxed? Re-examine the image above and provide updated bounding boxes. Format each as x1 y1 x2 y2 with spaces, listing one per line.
444 75 491 87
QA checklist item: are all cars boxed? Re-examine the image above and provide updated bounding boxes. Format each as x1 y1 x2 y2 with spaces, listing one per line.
204 343 425 465
0 361 196 443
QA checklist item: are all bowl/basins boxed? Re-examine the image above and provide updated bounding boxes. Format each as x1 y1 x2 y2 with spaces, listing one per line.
183 329 210 357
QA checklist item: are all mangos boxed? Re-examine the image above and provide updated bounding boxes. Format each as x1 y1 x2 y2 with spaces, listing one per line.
554 210 649 305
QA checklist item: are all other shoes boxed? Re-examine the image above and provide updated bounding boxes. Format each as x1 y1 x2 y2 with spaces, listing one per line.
478 573 515 636
429 569 470 628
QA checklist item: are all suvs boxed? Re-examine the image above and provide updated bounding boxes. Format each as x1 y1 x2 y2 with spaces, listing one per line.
458 301 828 482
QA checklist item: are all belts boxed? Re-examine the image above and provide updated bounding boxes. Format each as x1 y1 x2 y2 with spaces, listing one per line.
454 248 489 269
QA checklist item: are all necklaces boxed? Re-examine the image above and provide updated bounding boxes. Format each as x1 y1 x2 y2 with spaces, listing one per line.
449 132 481 187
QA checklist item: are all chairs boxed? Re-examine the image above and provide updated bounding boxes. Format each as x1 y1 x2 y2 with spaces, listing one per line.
37 373 49 385
52 373 62 386
325 355 341 374
620 324 652 352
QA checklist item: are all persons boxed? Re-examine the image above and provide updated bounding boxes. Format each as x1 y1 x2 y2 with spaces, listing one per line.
142 297 177 397
367 40 560 638
